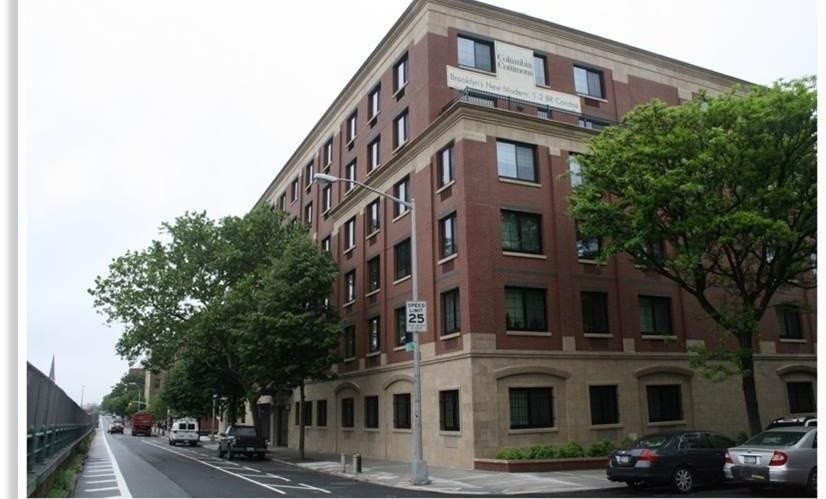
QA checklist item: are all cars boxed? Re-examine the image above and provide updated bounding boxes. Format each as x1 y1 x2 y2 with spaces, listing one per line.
106 414 124 434
167 417 200 448
767 416 816 428
605 430 746 493
725 426 818 497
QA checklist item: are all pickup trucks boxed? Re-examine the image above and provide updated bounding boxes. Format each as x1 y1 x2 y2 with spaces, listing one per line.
217 422 271 461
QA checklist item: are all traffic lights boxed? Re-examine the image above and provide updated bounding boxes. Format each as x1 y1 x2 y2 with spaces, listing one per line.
128 401 132 408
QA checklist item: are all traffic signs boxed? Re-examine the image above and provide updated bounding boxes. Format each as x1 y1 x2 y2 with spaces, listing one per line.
403 300 429 332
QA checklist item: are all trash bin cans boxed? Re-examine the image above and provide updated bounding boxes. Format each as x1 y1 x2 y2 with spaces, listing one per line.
352 453 361 474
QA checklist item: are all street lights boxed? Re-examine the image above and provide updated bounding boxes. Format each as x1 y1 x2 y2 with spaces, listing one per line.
313 170 431 486
129 381 140 413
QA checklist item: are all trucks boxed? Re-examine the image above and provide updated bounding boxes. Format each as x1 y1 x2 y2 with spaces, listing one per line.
132 413 153 436
